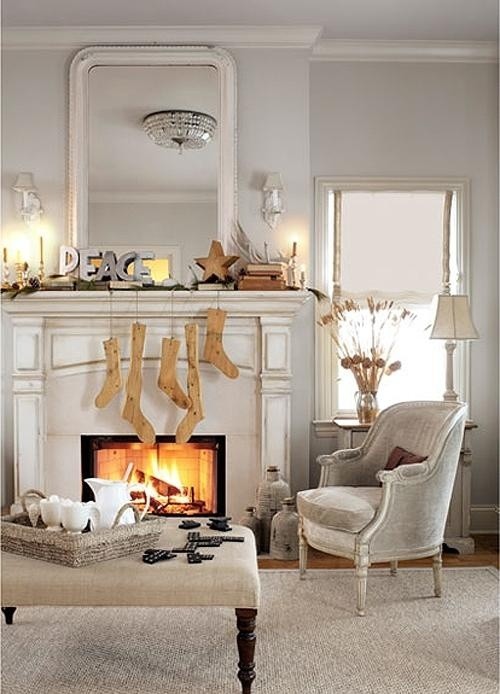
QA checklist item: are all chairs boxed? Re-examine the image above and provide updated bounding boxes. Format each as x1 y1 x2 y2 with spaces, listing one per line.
295 400 467 619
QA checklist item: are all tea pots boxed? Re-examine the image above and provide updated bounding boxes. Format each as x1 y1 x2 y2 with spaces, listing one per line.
83 478 149 531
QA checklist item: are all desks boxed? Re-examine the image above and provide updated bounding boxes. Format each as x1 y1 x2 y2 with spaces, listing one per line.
333 416 478 556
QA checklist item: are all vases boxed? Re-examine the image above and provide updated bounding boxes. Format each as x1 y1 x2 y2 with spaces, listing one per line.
355 390 378 424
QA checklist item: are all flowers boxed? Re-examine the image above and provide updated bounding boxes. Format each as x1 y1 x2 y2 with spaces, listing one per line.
315 296 418 421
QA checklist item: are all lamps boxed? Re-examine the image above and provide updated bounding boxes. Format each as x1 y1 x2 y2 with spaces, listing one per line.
141 110 218 156
13 172 43 224
262 171 290 227
430 294 479 403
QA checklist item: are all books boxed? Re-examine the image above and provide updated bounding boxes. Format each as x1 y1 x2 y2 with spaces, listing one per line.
79 279 143 291
196 281 238 291
242 261 286 280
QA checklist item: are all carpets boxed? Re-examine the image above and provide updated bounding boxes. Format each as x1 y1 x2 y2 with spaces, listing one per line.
2 567 498 694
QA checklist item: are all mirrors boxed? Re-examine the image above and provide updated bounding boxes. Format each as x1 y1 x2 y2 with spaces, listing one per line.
68 44 238 281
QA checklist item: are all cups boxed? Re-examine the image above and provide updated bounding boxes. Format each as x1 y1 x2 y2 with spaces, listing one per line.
40 499 100 534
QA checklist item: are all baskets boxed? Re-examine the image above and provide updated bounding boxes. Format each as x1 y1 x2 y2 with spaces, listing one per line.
1 490 165 566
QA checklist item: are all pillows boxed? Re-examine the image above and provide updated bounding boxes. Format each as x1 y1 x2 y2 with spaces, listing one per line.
380 447 430 489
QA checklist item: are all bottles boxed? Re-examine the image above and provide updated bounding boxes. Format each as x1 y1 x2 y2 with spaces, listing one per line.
241 465 297 562
